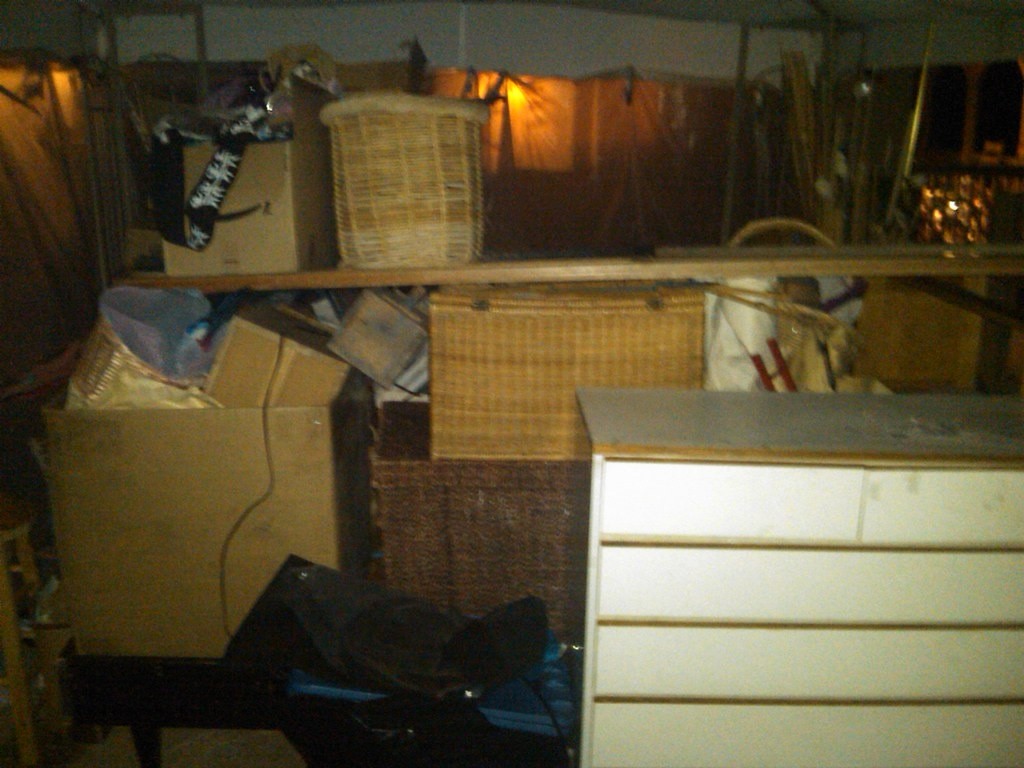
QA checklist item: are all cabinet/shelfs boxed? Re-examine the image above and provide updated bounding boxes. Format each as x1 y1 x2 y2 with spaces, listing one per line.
572 378 1024 768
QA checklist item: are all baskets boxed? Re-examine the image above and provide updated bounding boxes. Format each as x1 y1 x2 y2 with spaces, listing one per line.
323 92 488 265
428 285 708 462
68 317 221 409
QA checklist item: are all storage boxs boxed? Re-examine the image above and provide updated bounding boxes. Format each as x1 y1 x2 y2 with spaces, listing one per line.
149 142 330 277
38 300 376 662
